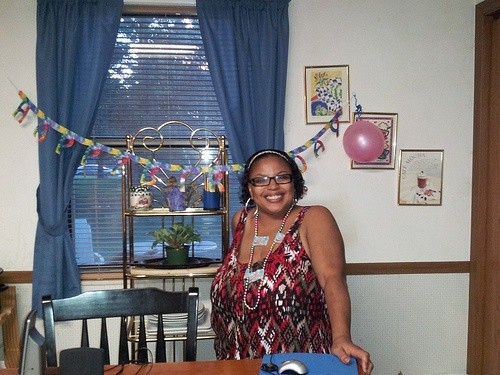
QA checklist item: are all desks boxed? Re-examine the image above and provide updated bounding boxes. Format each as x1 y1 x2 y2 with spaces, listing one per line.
0 351 363 375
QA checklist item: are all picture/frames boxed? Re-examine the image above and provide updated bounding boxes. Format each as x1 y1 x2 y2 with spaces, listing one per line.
351 111 398 171
303 64 351 125
396 146 445 207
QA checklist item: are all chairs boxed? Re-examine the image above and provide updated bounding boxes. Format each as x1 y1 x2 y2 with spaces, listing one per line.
42 285 201 366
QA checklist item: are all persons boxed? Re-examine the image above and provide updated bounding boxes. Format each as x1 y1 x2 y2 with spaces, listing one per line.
209 149 375 375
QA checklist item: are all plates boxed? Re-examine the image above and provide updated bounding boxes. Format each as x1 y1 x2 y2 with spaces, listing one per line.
130 256 223 269
135 298 215 340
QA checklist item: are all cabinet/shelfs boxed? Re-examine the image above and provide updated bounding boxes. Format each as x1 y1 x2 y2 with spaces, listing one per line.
120 125 232 361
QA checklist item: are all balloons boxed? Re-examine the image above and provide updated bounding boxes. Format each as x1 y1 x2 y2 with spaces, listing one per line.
342 120 385 163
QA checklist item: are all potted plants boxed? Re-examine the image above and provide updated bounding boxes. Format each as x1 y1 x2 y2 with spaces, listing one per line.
152 222 200 266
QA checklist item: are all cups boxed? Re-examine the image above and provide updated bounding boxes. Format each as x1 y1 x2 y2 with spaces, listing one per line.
130 185 152 211
204 170 221 210
417 175 431 188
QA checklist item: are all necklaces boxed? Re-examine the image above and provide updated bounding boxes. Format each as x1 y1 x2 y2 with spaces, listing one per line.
243 202 298 311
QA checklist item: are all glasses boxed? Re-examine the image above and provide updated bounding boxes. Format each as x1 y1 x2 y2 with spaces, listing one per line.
248 174 293 186
